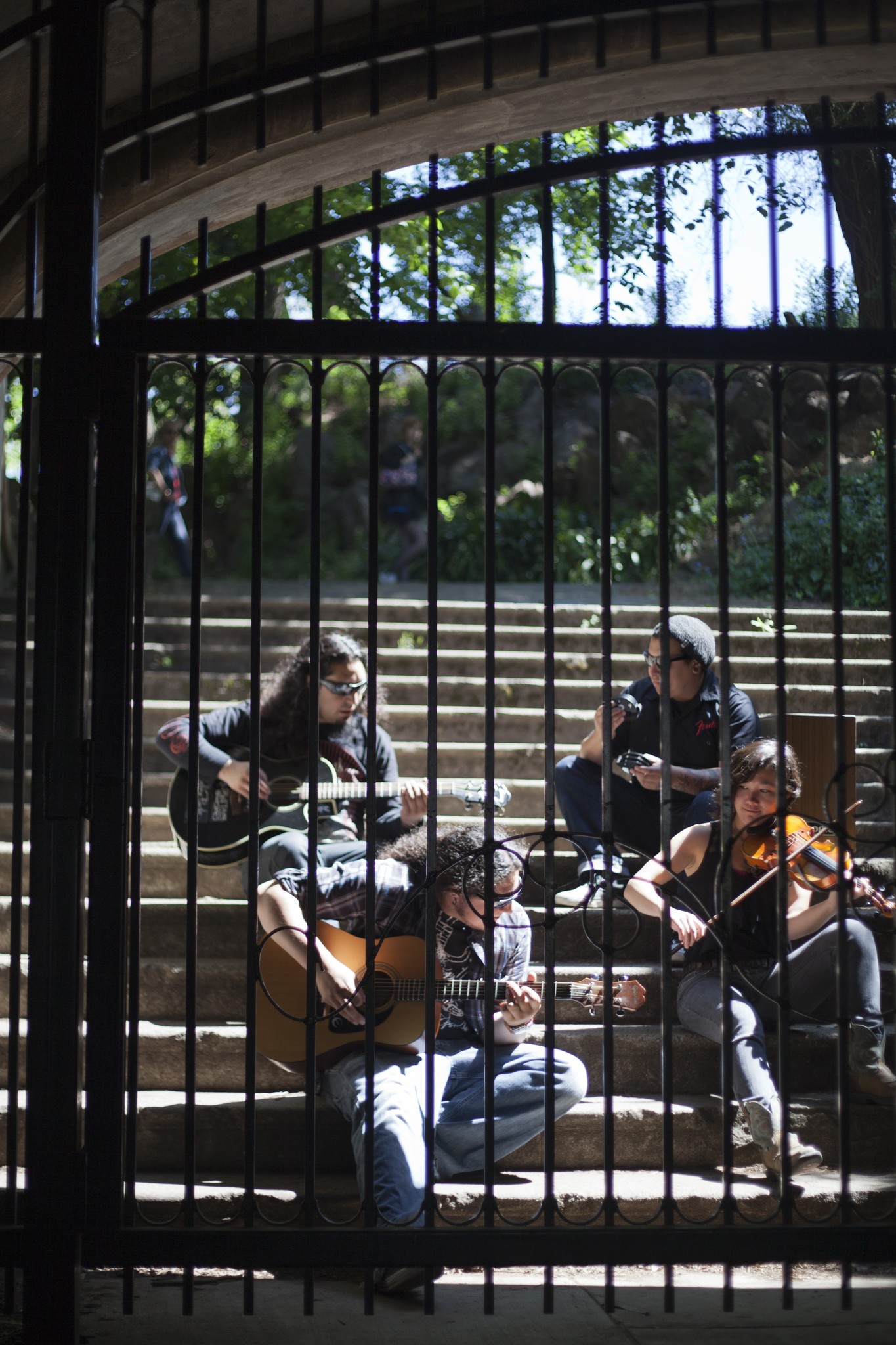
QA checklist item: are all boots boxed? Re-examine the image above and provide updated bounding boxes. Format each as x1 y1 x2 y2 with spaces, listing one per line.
848 1023 896 1106
739 1094 823 1177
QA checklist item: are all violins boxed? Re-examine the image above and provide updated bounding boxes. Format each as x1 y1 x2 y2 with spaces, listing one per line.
743 813 896 919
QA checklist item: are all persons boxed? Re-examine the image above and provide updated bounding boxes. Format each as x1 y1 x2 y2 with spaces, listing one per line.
374 414 428 589
146 418 192 591
555 614 762 907
255 825 588 1296
621 738 896 1177
154 630 427 894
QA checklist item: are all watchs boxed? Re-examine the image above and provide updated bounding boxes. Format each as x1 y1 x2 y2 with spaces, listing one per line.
502 1018 534 1036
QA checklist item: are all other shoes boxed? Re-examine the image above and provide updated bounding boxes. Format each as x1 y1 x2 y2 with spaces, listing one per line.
555 873 626 908
376 1266 446 1296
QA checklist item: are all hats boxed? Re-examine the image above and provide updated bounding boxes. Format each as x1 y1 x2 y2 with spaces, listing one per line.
654 614 716 668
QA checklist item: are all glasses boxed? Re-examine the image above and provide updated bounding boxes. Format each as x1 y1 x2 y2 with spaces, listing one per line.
321 680 367 696
642 649 685 670
477 876 524 909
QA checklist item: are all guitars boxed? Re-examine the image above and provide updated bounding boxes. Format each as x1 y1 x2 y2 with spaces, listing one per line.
168 739 512 870
253 922 647 1064
